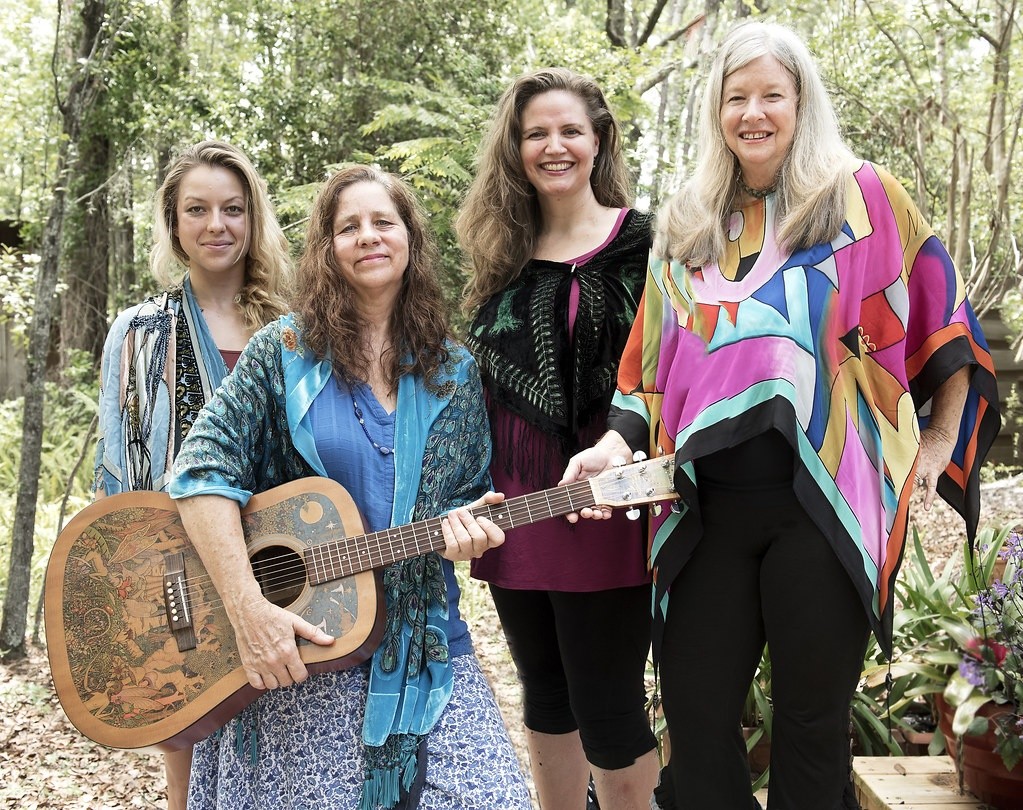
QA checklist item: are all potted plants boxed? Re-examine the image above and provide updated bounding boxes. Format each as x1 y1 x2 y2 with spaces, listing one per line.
847 630 907 759
743 643 776 794
855 519 1023 758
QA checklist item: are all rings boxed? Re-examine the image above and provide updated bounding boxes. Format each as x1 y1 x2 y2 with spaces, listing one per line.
913 473 924 487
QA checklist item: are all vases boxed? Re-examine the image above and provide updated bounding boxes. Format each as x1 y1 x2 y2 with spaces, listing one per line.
930 682 1023 810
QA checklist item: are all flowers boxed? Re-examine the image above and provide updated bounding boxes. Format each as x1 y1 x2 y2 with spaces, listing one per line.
961 531 1023 772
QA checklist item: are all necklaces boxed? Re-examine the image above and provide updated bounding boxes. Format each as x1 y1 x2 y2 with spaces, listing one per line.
734 155 782 200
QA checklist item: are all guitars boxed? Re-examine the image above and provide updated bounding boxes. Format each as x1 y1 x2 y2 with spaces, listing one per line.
42 449 682 751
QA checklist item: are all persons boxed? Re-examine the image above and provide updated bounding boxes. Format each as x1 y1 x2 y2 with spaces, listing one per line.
445 69 671 810
89 135 302 809
162 161 539 809
553 18 1004 810
